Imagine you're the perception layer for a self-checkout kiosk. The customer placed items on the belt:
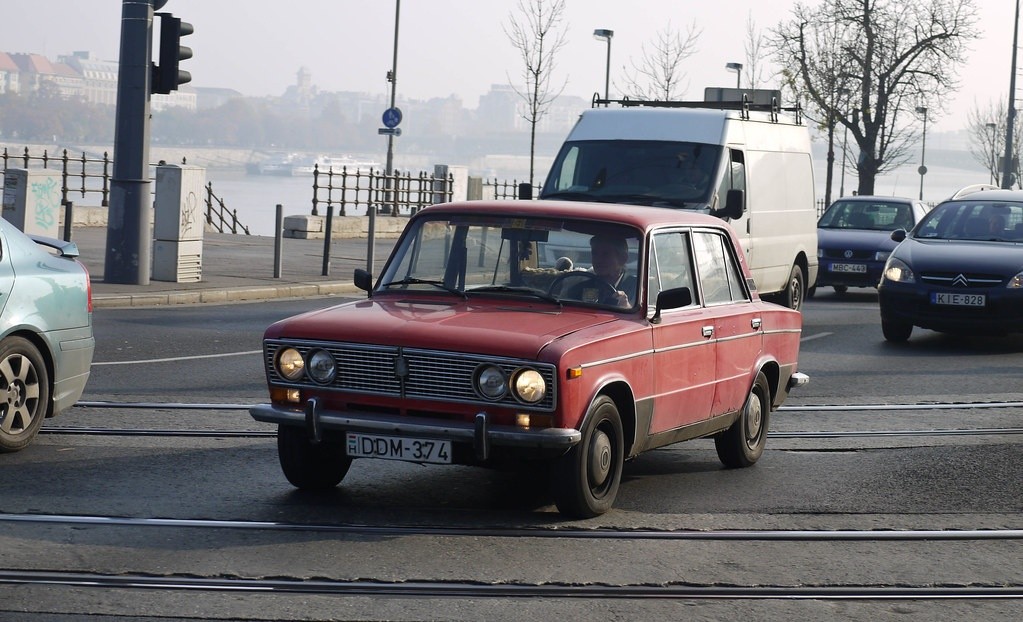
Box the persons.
[896,207,911,226]
[989,213,1005,233]
[567,235,637,308]
[685,158,711,189]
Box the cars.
[0,216,96,451]
[249,199,810,519]
[878,184,1023,342]
[817,195,931,299]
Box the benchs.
[516,266,666,308]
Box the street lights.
[984,123,997,187]
[915,105,927,198]
[594,28,614,105]
[834,87,851,195]
[724,63,744,89]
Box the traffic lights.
[154,13,193,95]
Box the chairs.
[965,218,989,238]
[846,212,869,227]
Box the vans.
[515,92,819,311]
